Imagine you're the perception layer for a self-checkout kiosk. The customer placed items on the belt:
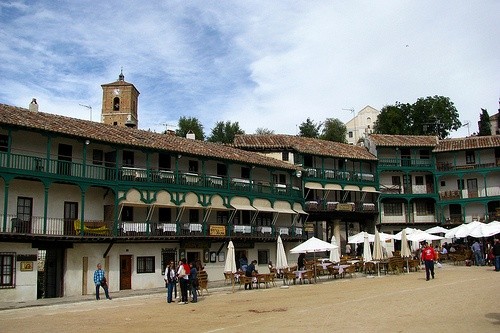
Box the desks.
[121,167,254,192]
[183,223,202,235]
[368,261,379,276]
[317,263,332,275]
[273,183,300,197]
[117,222,151,236]
[347,260,361,271]
[305,201,375,211]
[277,227,289,236]
[304,169,375,181]
[260,226,272,235]
[229,225,251,234]
[292,270,309,284]
[157,223,177,235]
[294,228,302,235]
[332,265,350,279]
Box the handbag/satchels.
[192,278,199,290]
[437,261,442,267]
[99,277,106,286]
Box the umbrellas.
[276,235,290,286]
[348,231,395,277]
[290,236,339,283]
[394,227,445,274]
[424,220,500,239]
[223,241,237,293]
[329,235,340,264]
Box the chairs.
[11,218,24,233]
[224,249,489,293]
[34,159,45,172]
[197,271,210,297]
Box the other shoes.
[190,300,197,303]
[168,300,175,303]
[426,278,429,281]
[432,275,434,278]
[178,301,188,305]
[97,297,100,300]
[107,296,111,299]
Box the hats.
[181,258,186,262]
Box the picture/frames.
[21,261,33,271]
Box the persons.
[440,234,500,272]
[421,242,438,281]
[345,243,362,256]
[296,252,308,270]
[245,260,259,290]
[93,263,111,301]
[165,257,199,305]
